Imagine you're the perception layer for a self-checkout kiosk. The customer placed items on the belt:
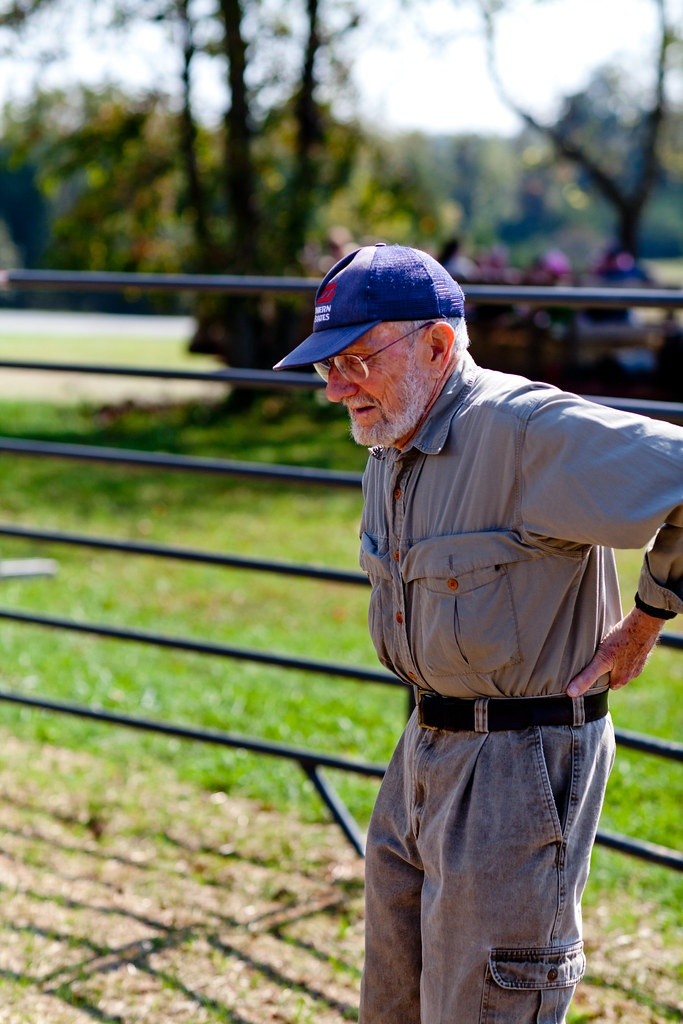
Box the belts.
[417,688,609,732]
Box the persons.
[271,242,682,1024]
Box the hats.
[272,243,464,371]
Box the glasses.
[312,321,434,384]
